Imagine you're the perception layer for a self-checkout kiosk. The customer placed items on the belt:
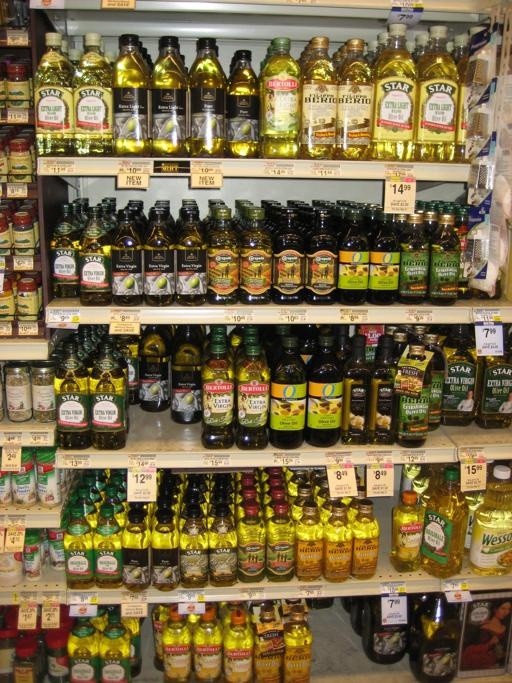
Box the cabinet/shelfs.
[1,333,66,611]
[1,8,68,339]
[36,149,512,606]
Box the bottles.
[264,488,291,522]
[422,333,444,430]
[385,324,426,364]
[33,33,74,156]
[151,36,189,158]
[389,491,424,574]
[236,502,266,583]
[88,341,126,450]
[112,34,150,157]
[272,207,305,303]
[76,468,111,528]
[408,594,461,683]
[12,638,39,683]
[0,629,18,683]
[287,470,310,506]
[234,345,270,451]
[67,617,100,683]
[223,610,254,683]
[49,204,79,299]
[323,502,352,583]
[475,324,512,429]
[254,606,284,683]
[223,601,250,628]
[121,508,151,592]
[11,451,37,507]
[278,598,308,625]
[200,332,233,362]
[263,478,287,504]
[319,491,342,523]
[348,486,366,522]
[367,335,397,444]
[352,499,379,580]
[151,468,184,528]
[68,197,468,235]
[429,214,460,305]
[19,205,40,248]
[151,604,170,662]
[176,211,206,306]
[0,277,15,322]
[449,34,468,64]
[78,206,112,308]
[162,611,192,683]
[93,504,123,589]
[0,214,12,257]
[63,507,95,590]
[48,519,67,570]
[6,63,30,109]
[336,39,375,160]
[235,334,266,364]
[206,472,235,528]
[368,211,400,305]
[31,363,56,423]
[179,504,209,589]
[107,336,130,434]
[3,362,32,422]
[206,209,239,305]
[291,484,313,522]
[179,473,210,529]
[12,211,35,256]
[412,31,429,64]
[420,467,468,578]
[208,503,237,587]
[239,207,272,304]
[54,343,89,450]
[266,503,296,583]
[469,464,512,576]
[17,278,39,322]
[416,25,460,163]
[236,474,261,503]
[0,144,8,183]
[188,37,227,158]
[200,342,235,450]
[16,132,37,177]
[192,605,223,683]
[151,508,180,592]
[99,615,131,683]
[22,622,47,676]
[340,334,371,446]
[371,25,419,162]
[112,210,143,307]
[305,334,344,447]
[22,535,42,582]
[236,489,264,521]
[73,32,113,157]
[120,615,142,678]
[30,526,47,563]
[58,39,70,60]
[361,596,406,664]
[137,325,171,413]
[0,453,13,508]
[441,323,479,428]
[455,210,472,300]
[300,35,338,161]
[315,477,328,507]
[269,336,307,451]
[170,324,202,425]
[45,630,69,683]
[295,501,323,579]
[304,210,339,305]
[144,210,175,307]
[373,30,390,62]
[259,37,300,160]
[455,25,487,163]
[399,213,429,305]
[36,449,62,507]
[224,50,259,158]
[394,345,427,448]
[263,469,286,491]
[117,334,139,404]
[73,324,129,377]
[8,138,33,183]
[284,605,312,683]
[336,214,369,305]
[104,469,127,528]
